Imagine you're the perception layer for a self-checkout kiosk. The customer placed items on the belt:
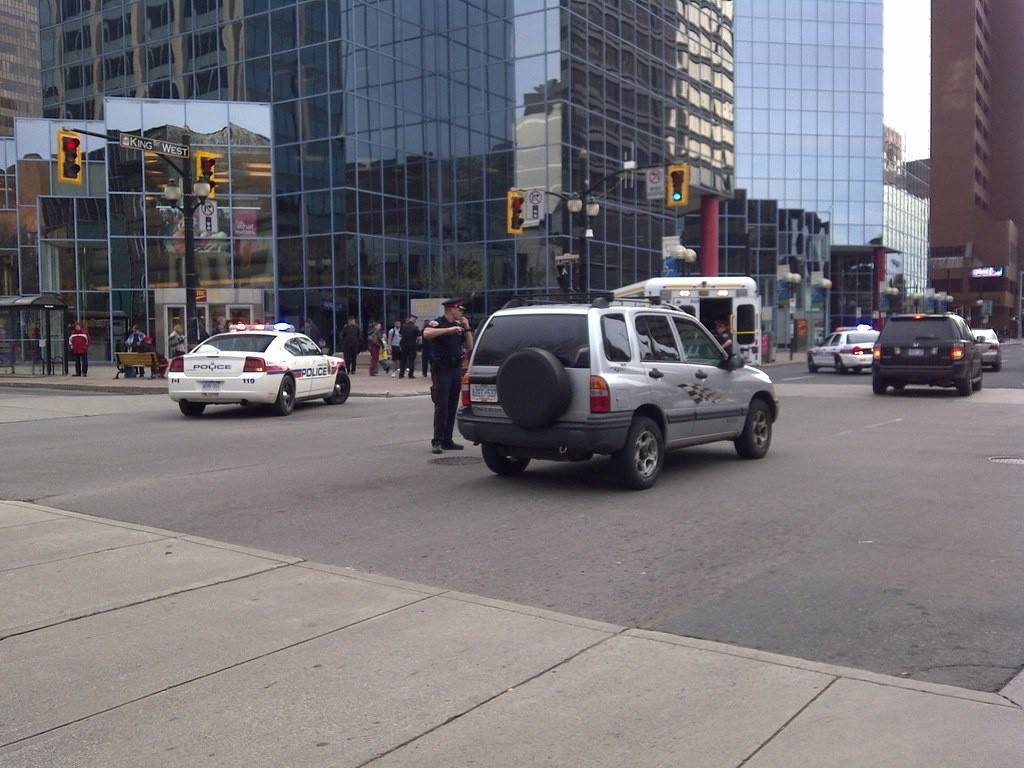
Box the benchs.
[33,356,64,376]
[113,352,164,380]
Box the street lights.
[566,192,600,304]
[784,273,801,361]
[671,245,697,276]
[913,293,922,314]
[163,175,210,353]
[933,294,942,315]
[946,295,954,311]
[819,279,832,340]
[885,287,899,315]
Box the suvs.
[872,311,986,397]
[456,292,782,490]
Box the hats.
[143,336,153,341]
[715,319,728,328]
[442,297,466,310]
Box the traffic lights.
[667,165,690,207]
[196,150,216,199]
[57,131,82,184]
[507,191,525,235]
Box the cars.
[807,327,881,376]
[168,330,351,416]
[971,329,1004,372]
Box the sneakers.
[442,441,464,450]
[432,445,442,454]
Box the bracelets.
[465,327,471,332]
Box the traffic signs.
[120,133,189,159]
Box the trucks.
[607,276,761,367]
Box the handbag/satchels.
[367,334,380,348]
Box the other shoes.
[399,376,405,379]
[409,376,415,379]
[386,367,390,373]
[347,371,350,374]
[72,374,81,376]
[351,370,355,374]
[83,374,87,377]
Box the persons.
[125,325,169,379]
[423,297,473,454]
[68,322,92,377]
[368,314,432,379]
[303,319,323,352]
[160,207,235,252]
[989,267,1001,276]
[198,316,273,345]
[340,316,363,374]
[168,324,187,358]
[716,322,733,355]
